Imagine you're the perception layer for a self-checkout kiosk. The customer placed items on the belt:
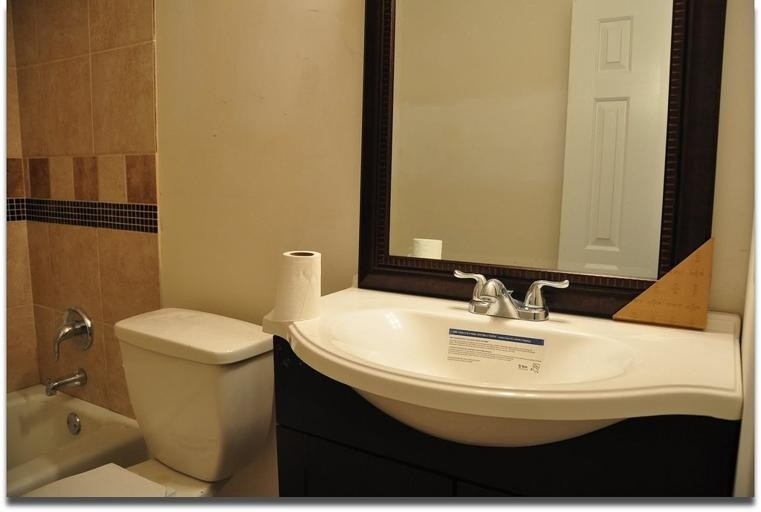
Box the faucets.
[44,368,87,397]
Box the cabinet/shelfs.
[270,346,740,499]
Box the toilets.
[22,304,276,499]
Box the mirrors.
[356,1,729,334]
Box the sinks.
[480,278,519,319]
[330,308,635,448]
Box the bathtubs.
[7,382,149,497]
